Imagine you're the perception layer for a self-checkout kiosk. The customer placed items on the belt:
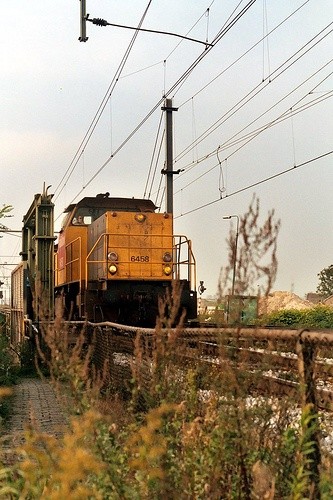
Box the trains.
[51,191,206,335]
